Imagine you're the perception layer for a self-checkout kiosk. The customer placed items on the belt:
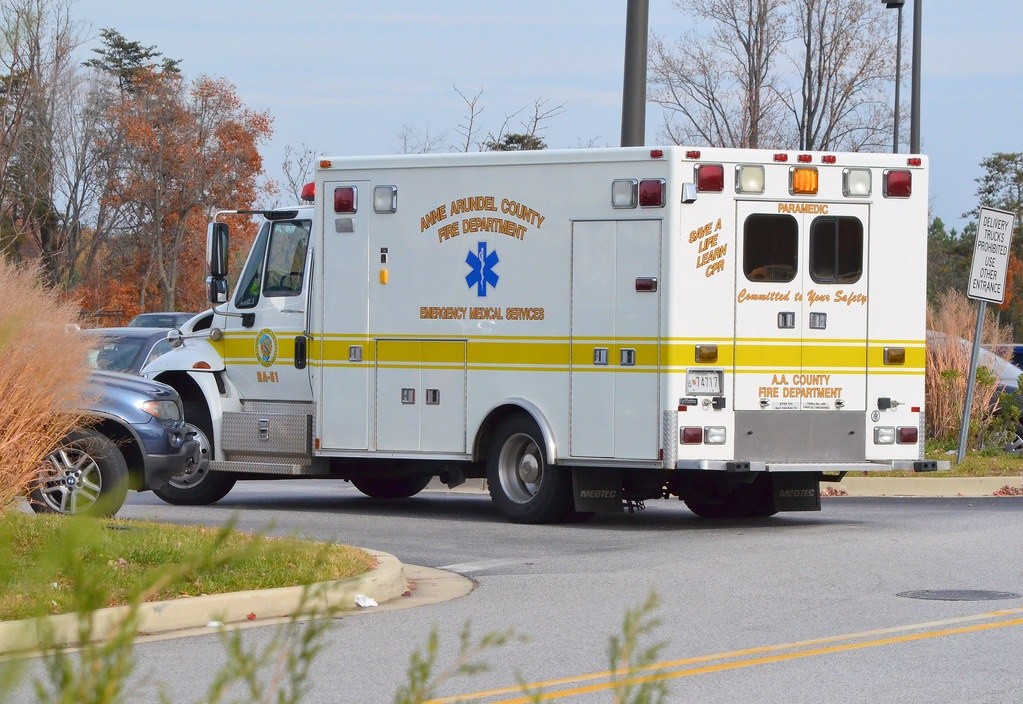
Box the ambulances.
[140,145,930,507]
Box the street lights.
[883,0,906,151]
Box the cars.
[981,344,1022,370]
[928,331,1023,455]
[132,312,200,330]
[73,326,177,377]
[26,369,190,516]
